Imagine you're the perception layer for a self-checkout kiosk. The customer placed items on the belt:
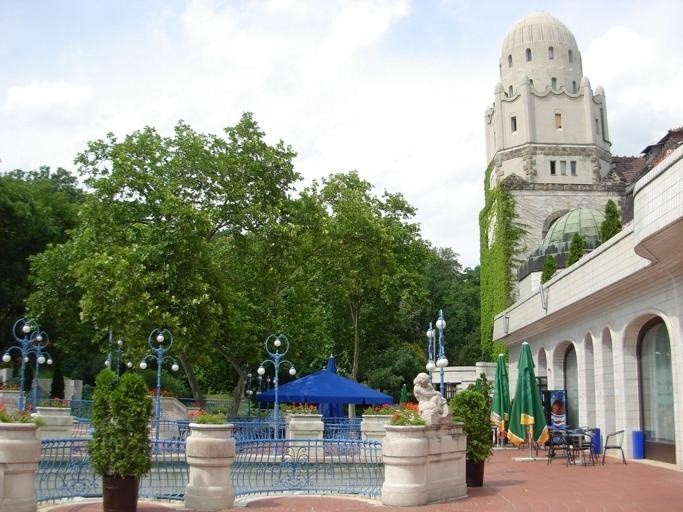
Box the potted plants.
[93,370,152,512]
[449,372,492,487]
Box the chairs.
[547,426,626,467]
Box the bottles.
[551,392,566,431]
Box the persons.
[412,372,450,423]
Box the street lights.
[138,326,180,453]
[245,368,253,417]
[434,307,449,397]
[424,319,438,383]
[256,331,296,455]
[23,329,54,408]
[0,317,46,413]
[101,338,134,376]
[265,373,271,414]
[255,375,263,415]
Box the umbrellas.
[397,382,408,404]
[253,367,394,415]
[313,351,347,437]
[475,377,481,392]
[506,340,551,462]
[490,351,518,451]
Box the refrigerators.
[540,389,567,445]
